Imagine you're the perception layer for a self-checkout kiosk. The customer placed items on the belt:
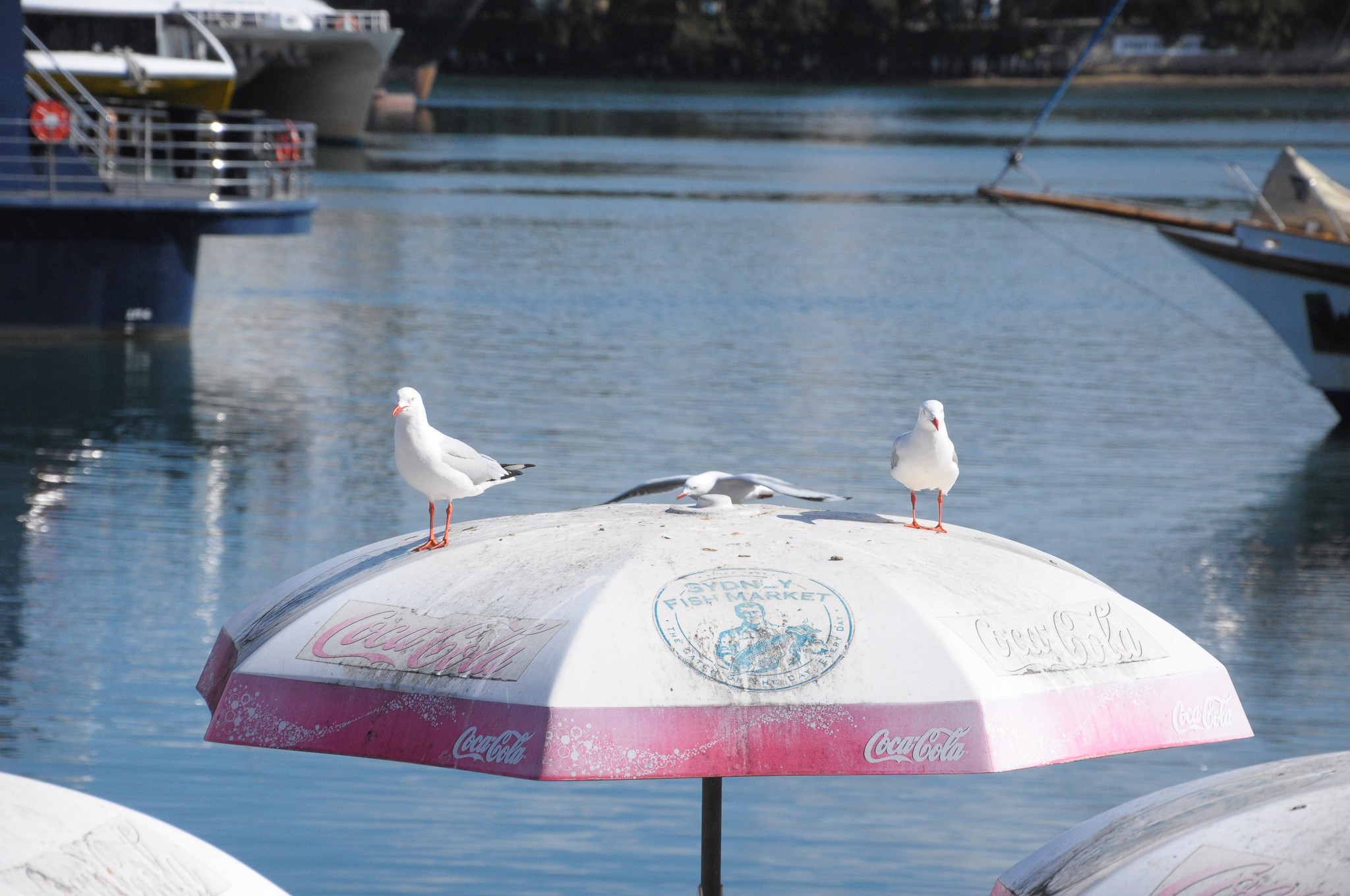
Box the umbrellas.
[197,497,1254,896]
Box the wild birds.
[597,471,852,503]
[392,387,536,551]
[890,401,960,532]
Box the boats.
[20,0,406,151]
[979,0,1350,446]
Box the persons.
[267,109,300,200]
